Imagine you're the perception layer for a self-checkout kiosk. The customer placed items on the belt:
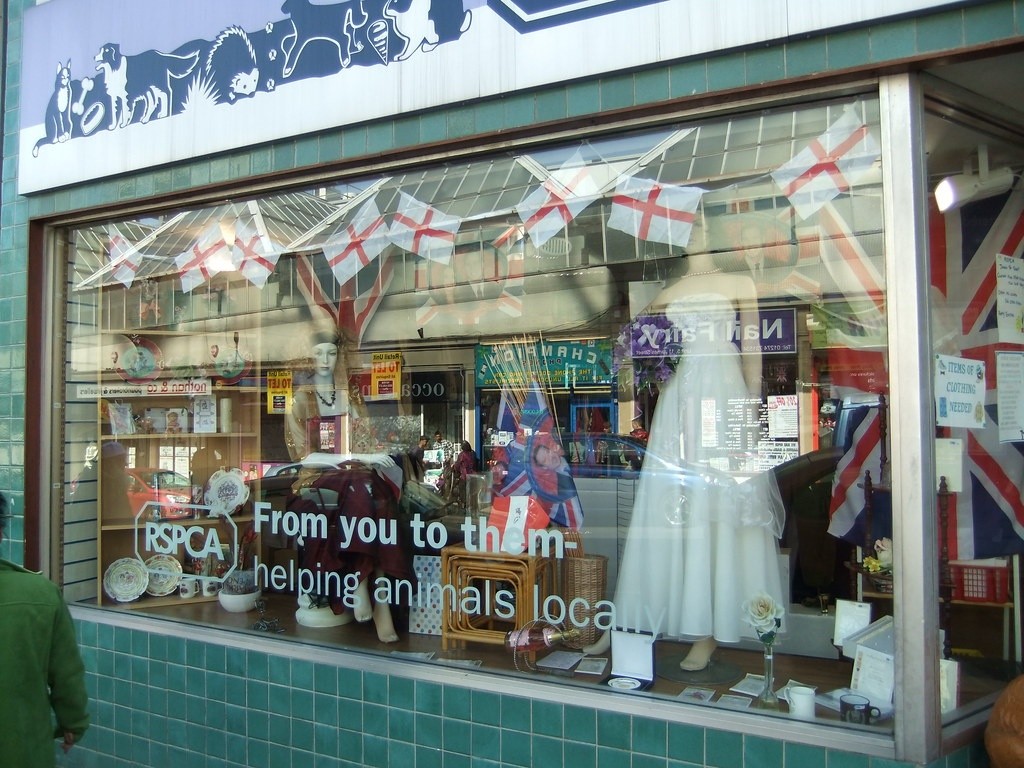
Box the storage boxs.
[948,561,1010,603]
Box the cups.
[840,694,881,726]
[203,577,223,597]
[179,577,199,599]
[784,686,816,718]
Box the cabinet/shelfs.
[95,238,260,608]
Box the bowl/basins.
[218,588,262,613]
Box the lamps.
[933,143,1015,214]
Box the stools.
[441,541,558,665]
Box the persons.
[581,200,789,670]
[437,248,503,312]
[269,318,402,645]
[432,431,454,468]
[534,443,578,498]
[572,416,649,472]
[491,461,508,485]
[409,436,428,465]
[0,494,89,768]
[452,440,479,506]
[720,223,786,282]
[166,412,181,435]
[71,442,134,539]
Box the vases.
[759,648,778,703]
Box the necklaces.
[316,377,336,406]
[681,267,722,277]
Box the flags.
[174,223,235,294]
[501,382,585,530]
[814,191,1023,562]
[411,224,524,324]
[106,223,142,289]
[606,174,702,248]
[231,220,284,289]
[515,151,603,248]
[385,194,462,266]
[322,199,392,286]
[488,395,518,505]
[769,110,881,221]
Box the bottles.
[504,627,582,654]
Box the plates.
[144,553,183,596]
[203,468,250,517]
[103,557,148,602]
[112,336,165,387]
[204,341,253,384]
[608,677,640,691]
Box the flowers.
[740,595,784,643]
[613,313,683,386]
